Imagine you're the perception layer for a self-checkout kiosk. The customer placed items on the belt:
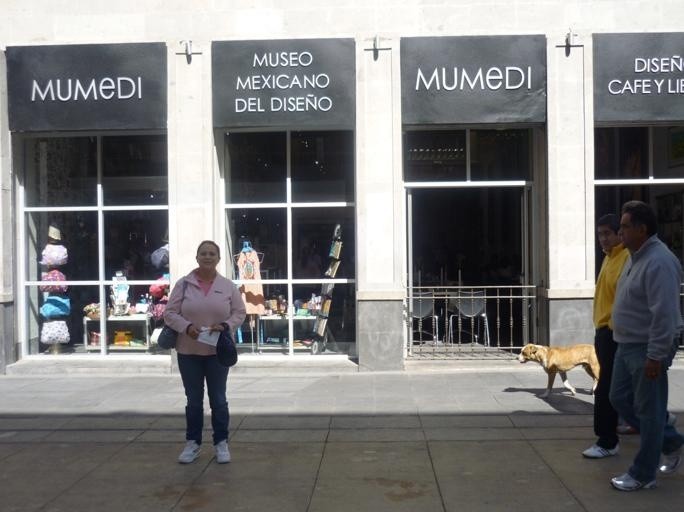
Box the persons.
[581,215,678,458]
[608,199,683,492]
[163,240,247,465]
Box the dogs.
[516,342,602,401]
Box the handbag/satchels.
[151,244,169,344]
[217,324,237,366]
[39,245,70,343]
[159,325,178,349]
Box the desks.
[256,312,318,354]
[82,312,154,354]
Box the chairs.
[412,292,439,347]
[448,290,490,347]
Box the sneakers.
[611,473,655,492]
[660,447,681,474]
[582,445,618,458]
[667,414,677,428]
[214,439,230,463]
[179,440,202,463]
